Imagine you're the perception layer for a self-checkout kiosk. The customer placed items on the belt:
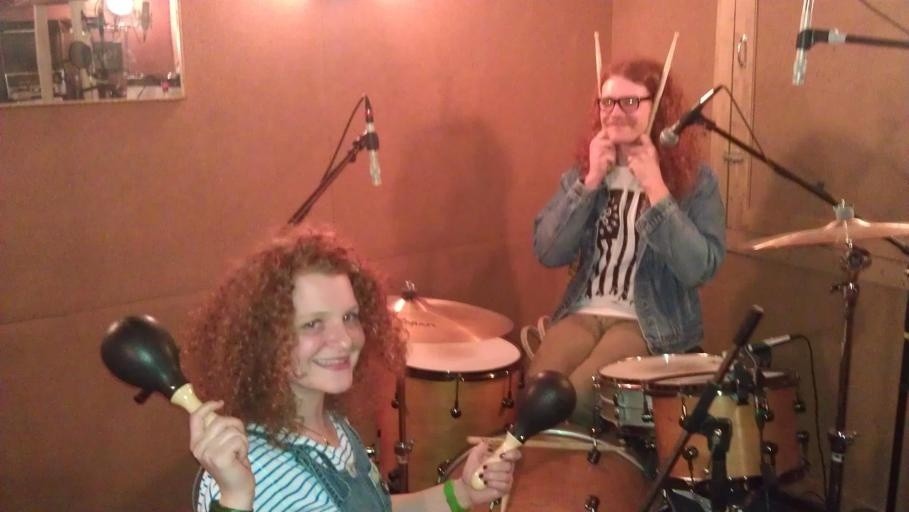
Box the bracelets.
[442,479,467,512]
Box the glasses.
[595,94,652,112]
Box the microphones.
[791,0,815,87]
[364,94,383,187]
[747,334,803,352]
[659,83,724,149]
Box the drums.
[651,371,802,486]
[438,425,674,512]
[379,338,522,495]
[593,353,734,448]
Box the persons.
[514,53,730,439]
[180,227,522,512]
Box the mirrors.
[1,0,184,108]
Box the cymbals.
[384,296,513,342]
[745,216,903,250]
